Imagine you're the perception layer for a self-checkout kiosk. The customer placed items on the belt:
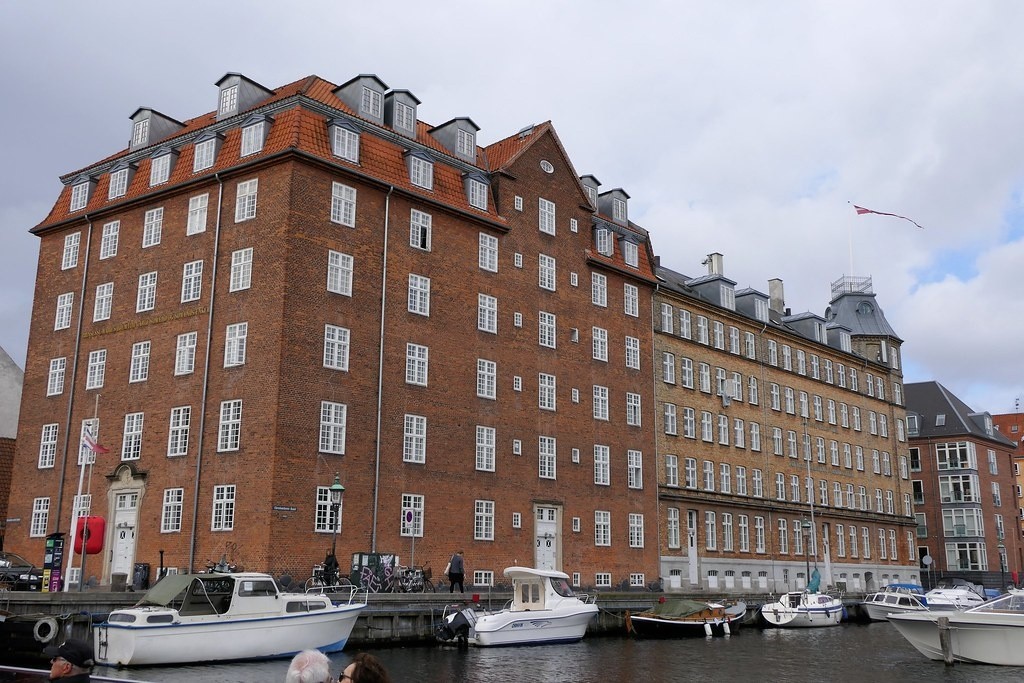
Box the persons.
[43,638,94,683]
[336,653,392,683]
[448,550,466,593]
[320,548,341,593]
[286,648,334,683]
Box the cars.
[0,551,64,592]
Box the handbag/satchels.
[443,555,454,576]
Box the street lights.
[801,515,812,587]
[327,471,346,555]
[996,538,1007,594]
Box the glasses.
[338,672,354,682]
[49,655,65,662]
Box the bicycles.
[408,561,436,593]
[193,559,236,592]
[304,564,353,593]
[392,564,425,593]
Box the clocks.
[859,302,872,315]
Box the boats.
[90,573,368,664]
[0,608,117,658]
[925,577,985,611]
[885,588,1024,666]
[436,566,599,646]
[626,600,747,635]
[762,588,849,626]
[858,584,930,620]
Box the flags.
[82,427,110,454]
[853,204,925,228]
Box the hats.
[42,638,96,669]
